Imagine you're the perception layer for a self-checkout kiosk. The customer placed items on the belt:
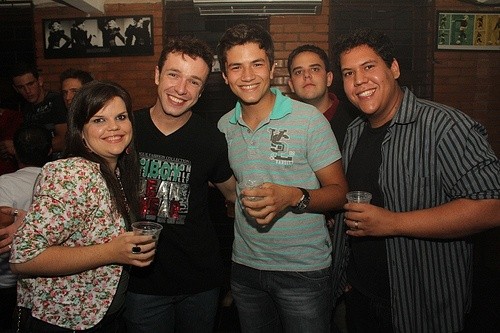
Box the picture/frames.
[437,12,500,51]
[42,14,154,59]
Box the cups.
[131,221,163,261]
[238,178,264,219]
[346,191,372,230]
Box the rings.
[133,243,141,254]
[355,220,359,229]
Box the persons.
[46,14,154,51]
[212,22,351,331]
[0,118,53,328]
[9,63,67,170]
[117,36,243,332]
[8,81,154,332]
[328,28,499,332]
[287,45,361,164]
[55,68,96,156]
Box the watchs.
[291,185,312,213]
